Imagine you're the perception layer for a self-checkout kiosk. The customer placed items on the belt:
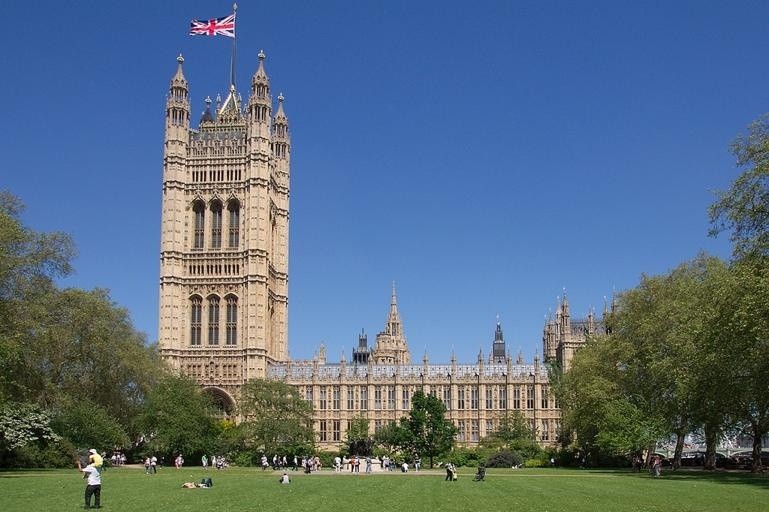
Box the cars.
[651,451,769,466]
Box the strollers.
[472,463,485,481]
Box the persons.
[77,454,102,510]
[652,455,661,477]
[149,455,158,474]
[202,454,229,470]
[452,467,457,481]
[142,455,150,474]
[278,473,292,485]
[175,453,185,472]
[85,449,103,474]
[180,477,212,489]
[110,452,128,465]
[445,458,452,481]
[261,452,423,475]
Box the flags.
[188,15,234,38]
